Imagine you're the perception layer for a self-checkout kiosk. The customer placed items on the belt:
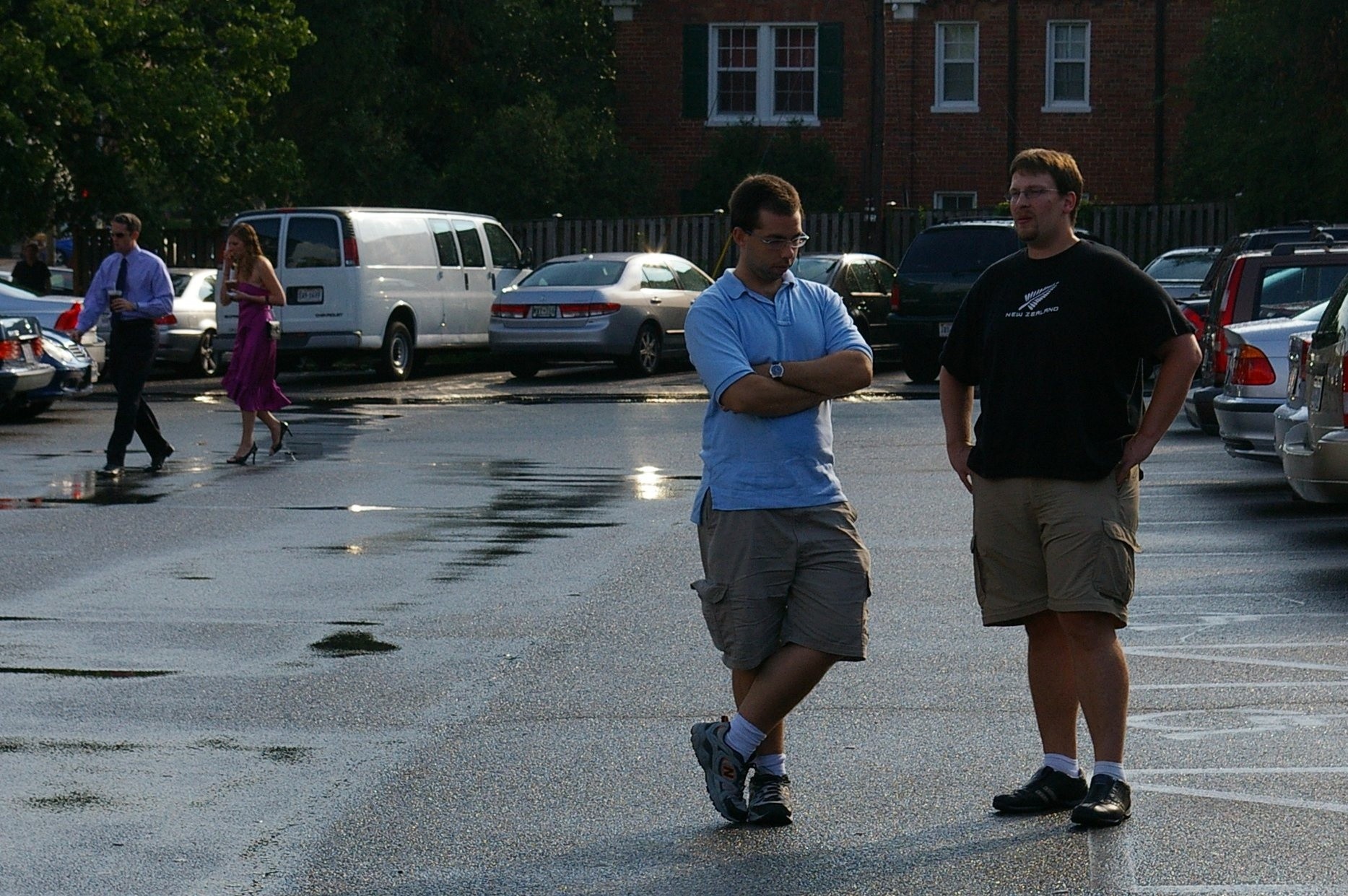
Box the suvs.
[1140,220,1348,505]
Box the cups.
[225,280,236,292]
[107,290,122,312]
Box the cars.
[789,252,898,366]
[488,252,715,378]
[0,265,112,422]
[153,268,219,377]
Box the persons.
[220,223,293,464]
[70,214,175,477]
[684,174,874,824]
[11,233,51,294]
[939,149,1203,824]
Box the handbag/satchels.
[266,296,281,340]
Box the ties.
[111,258,127,331]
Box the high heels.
[268,421,293,456]
[226,441,257,465]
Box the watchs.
[769,361,783,381]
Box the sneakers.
[745,774,794,826]
[1070,774,1133,827]
[690,715,755,822]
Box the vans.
[212,207,534,381]
[886,219,1106,383]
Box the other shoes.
[95,463,125,478]
[146,444,173,473]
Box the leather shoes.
[992,764,1088,817]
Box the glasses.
[740,230,810,252]
[110,230,131,239]
[1003,184,1062,199]
